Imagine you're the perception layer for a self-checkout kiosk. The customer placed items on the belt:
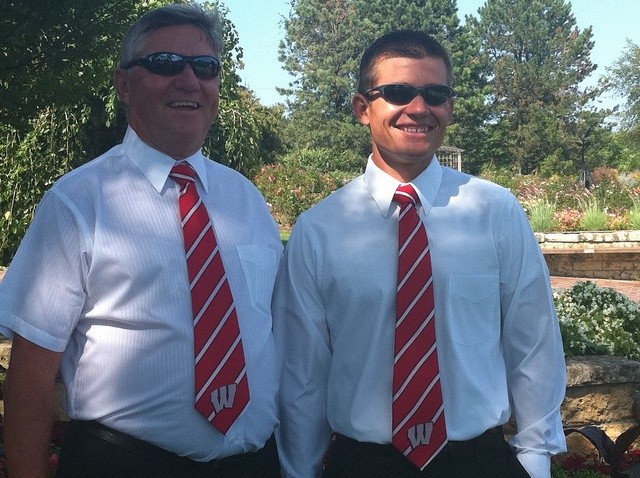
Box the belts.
[336,427,504,460]
[83,420,269,478]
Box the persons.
[1,5,285,476]
[269,28,569,478]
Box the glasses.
[120,51,222,79]
[363,82,456,106]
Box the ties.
[169,164,250,435]
[392,184,448,470]
